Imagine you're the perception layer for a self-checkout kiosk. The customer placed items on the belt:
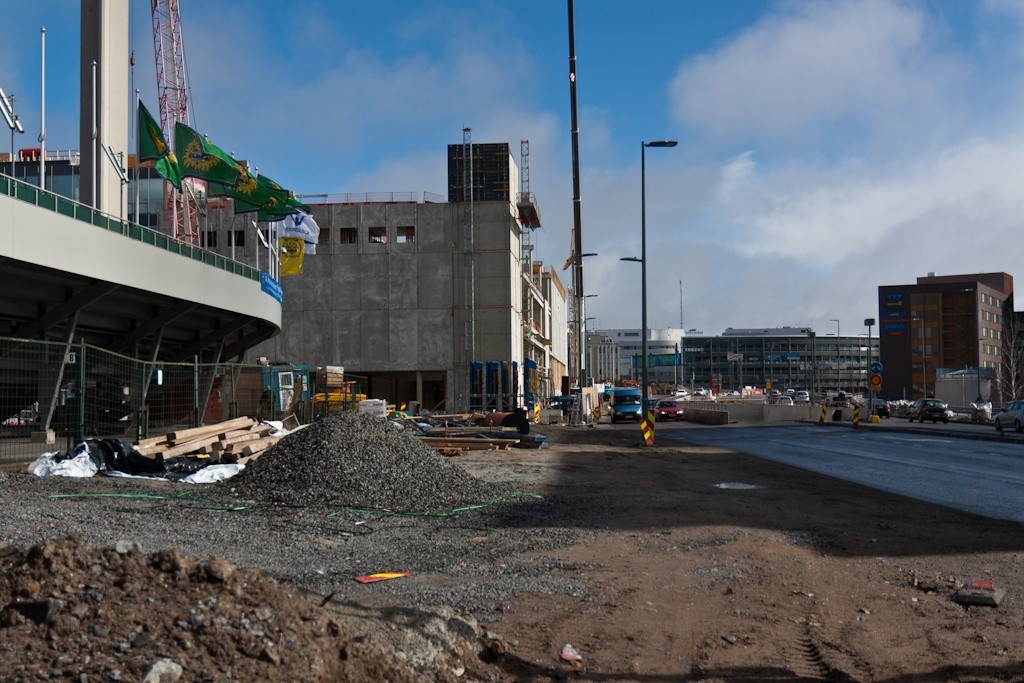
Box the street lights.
[829,319,841,397]
[705,338,713,374]
[620,137,680,439]
[960,287,981,400]
[808,332,816,407]
[863,318,876,415]
[913,316,927,399]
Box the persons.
[974,392,986,404]
[928,391,935,398]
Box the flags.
[138,98,181,190]
[236,165,320,277]
[175,120,241,187]
[207,139,277,208]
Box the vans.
[607,385,643,424]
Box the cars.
[671,389,677,396]
[906,398,949,424]
[866,399,891,419]
[992,400,1024,434]
[675,388,687,397]
[768,388,855,411]
[695,387,707,397]
[653,399,686,422]
